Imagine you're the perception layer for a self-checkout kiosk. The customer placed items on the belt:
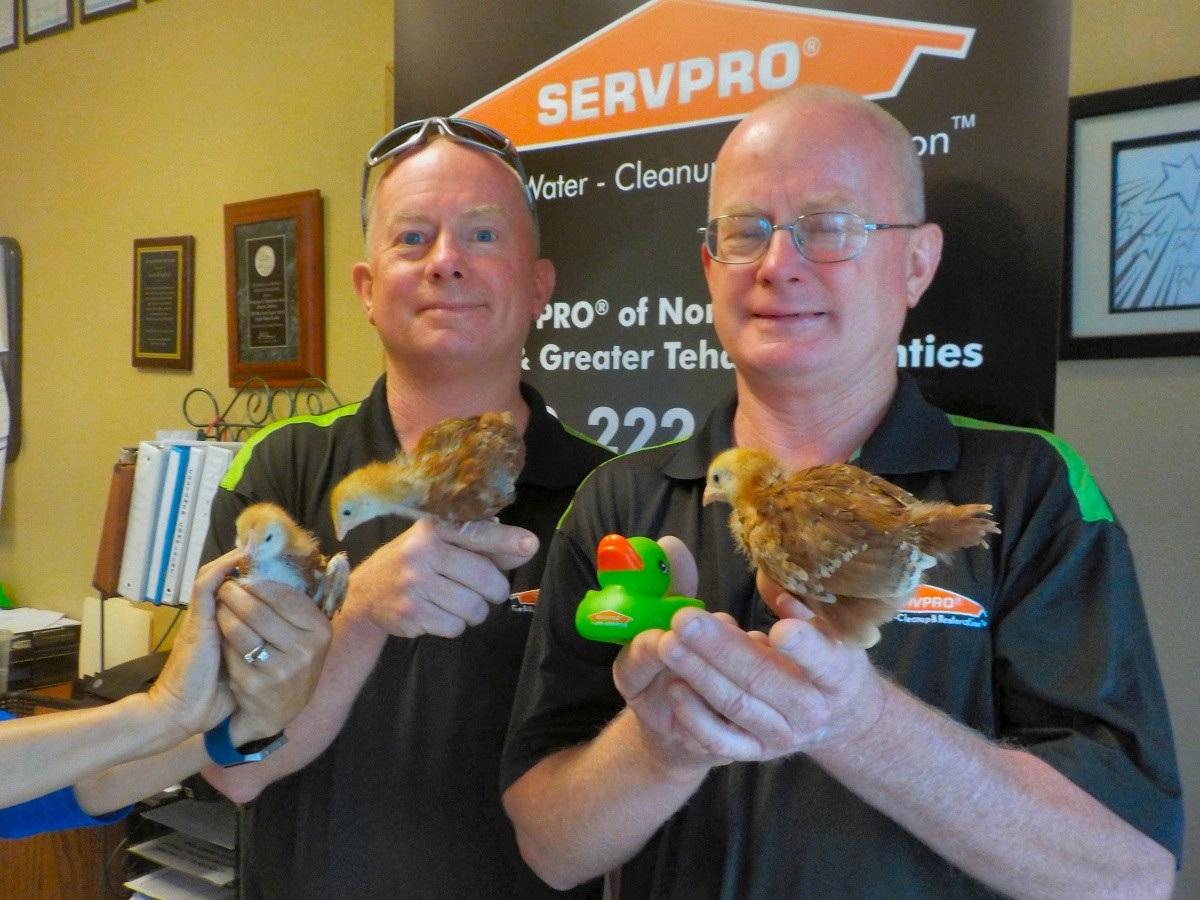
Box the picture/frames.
[1058,75,1200,359]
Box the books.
[93,435,249,607]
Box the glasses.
[359,115,539,243]
[695,211,918,264]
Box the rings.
[243,641,269,664]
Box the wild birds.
[234,501,350,618]
[701,448,1001,605]
[332,410,524,542]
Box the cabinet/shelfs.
[122,798,258,900]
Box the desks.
[0,682,132,900]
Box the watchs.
[204,714,290,768]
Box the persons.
[501,85,1184,900]
[0,548,333,838]
[197,116,623,900]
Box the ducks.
[575,535,701,649]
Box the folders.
[178,442,247,607]
[115,441,171,605]
